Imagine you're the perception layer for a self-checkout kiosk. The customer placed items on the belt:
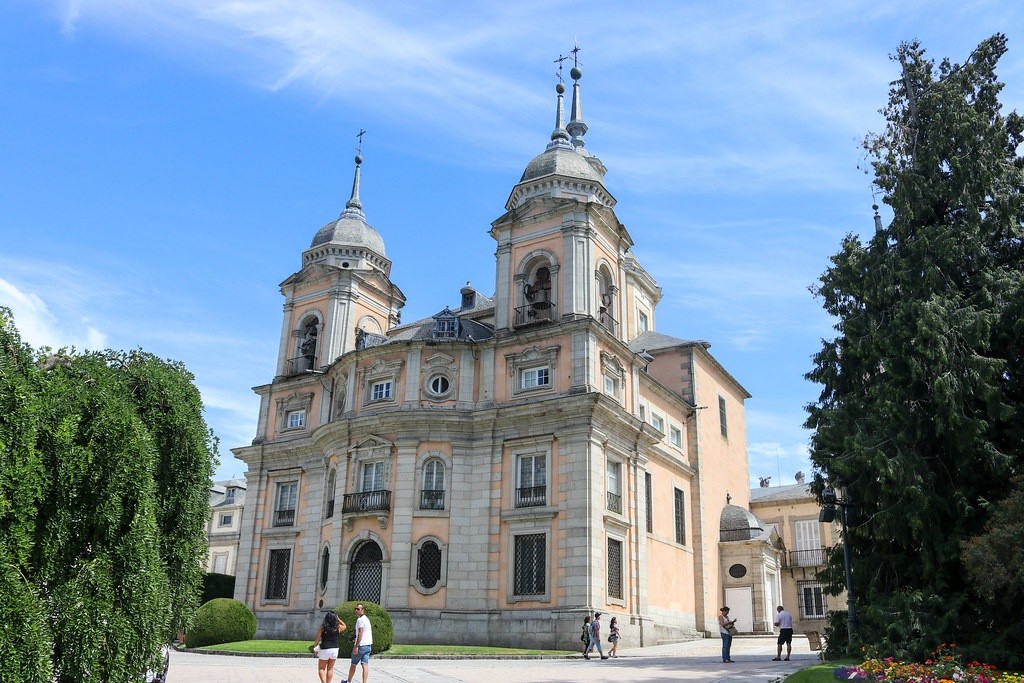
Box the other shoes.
[724,659,735,663]
[784,658,789,661]
[772,657,781,661]
[608,652,611,657]
[584,654,590,660]
[601,656,609,659]
[613,655,618,658]
[341,680,347,683]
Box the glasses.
[355,608,360,610]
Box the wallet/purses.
[313,644,321,657]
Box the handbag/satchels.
[581,634,585,641]
[608,636,612,642]
[727,626,738,636]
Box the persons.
[582,616,591,655]
[607,617,621,658]
[772,606,793,661]
[314,611,346,683]
[341,603,372,683]
[719,607,737,662]
[584,612,609,660]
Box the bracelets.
[355,645,360,647]
[338,620,339,621]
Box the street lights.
[818,485,855,649]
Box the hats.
[595,612,601,617]
